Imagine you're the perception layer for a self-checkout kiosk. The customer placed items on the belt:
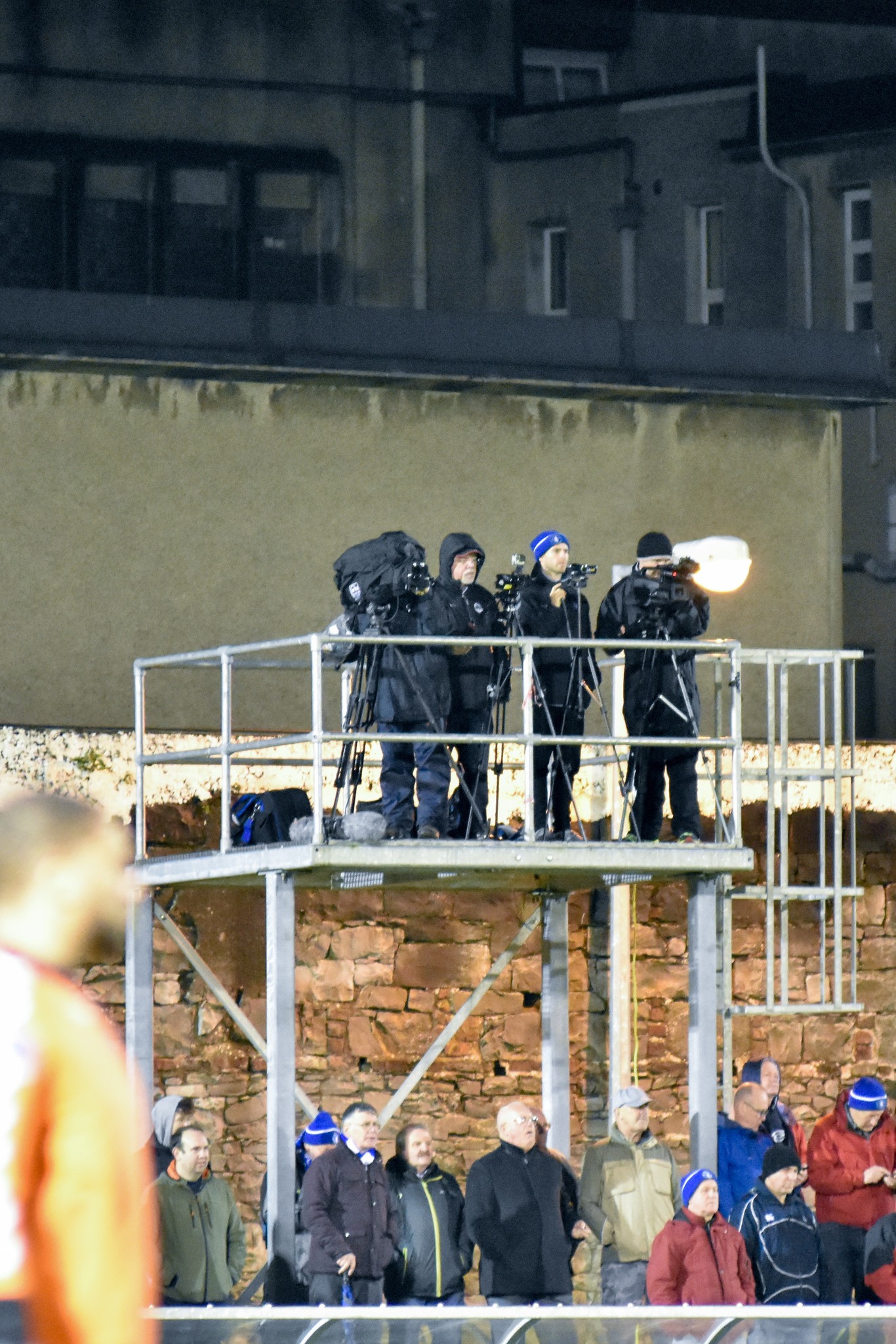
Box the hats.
[636,532,672,561]
[530,530,570,563]
[762,1143,802,1178]
[303,1110,341,1146]
[681,1170,718,1208]
[847,1077,888,1111]
[456,548,483,557]
[613,1087,653,1112]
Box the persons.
[513,531,602,842]
[367,531,509,839]
[594,531,714,843]
[145,1056,896,1344]
[0,796,162,1344]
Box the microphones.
[288,809,388,844]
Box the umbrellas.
[342,1268,356,1343]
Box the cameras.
[569,563,597,575]
[496,554,533,590]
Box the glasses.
[501,1115,539,1129]
[744,1101,769,1118]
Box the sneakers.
[622,832,659,843]
[676,832,701,843]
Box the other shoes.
[418,825,439,839]
[384,825,406,839]
[535,828,554,840]
[548,830,584,841]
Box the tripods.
[326,574,731,845]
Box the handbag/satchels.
[230,787,312,847]
